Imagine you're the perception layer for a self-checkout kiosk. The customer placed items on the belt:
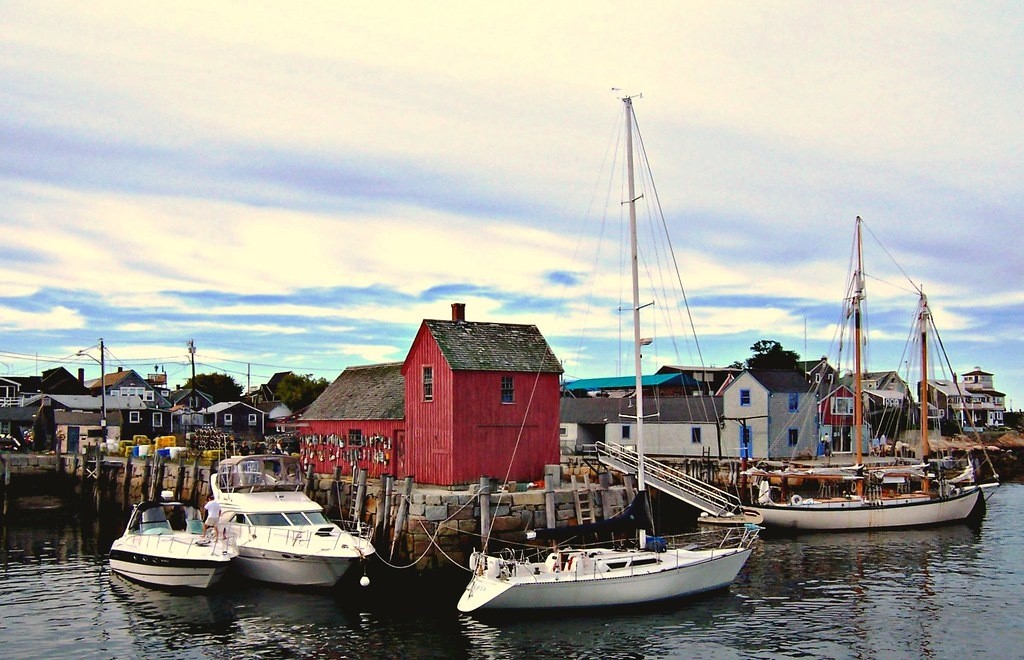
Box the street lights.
[76,353,107,459]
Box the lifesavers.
[851,482,857,493]
[792,494,803,507]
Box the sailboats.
[738,216,1008,534]
[453,88,760,613]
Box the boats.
[108,490,237,590]
[211,454,376,588]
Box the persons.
[872,434,903,456]
[199,495,221,543]
[227,431,300,479]
[822,432,833,457]
